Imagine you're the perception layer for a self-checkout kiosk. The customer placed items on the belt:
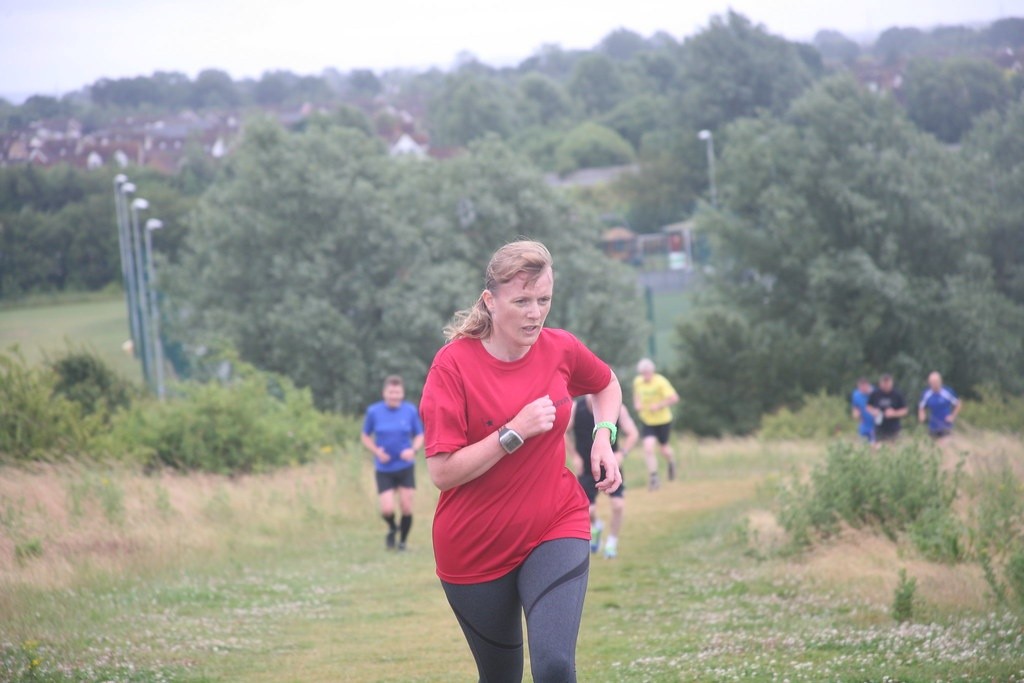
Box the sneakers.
[605,534,618,558]
[650,472,659,490]
[399,542,406,549]
[387,526,398,545]
[669,461,676,480]
[590,520,602,553]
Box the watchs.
[499,425,524,455]
[590,423,618,445]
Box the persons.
[360,375,424,550]
[564,388,638,556]
[632,358,677,488]
[417,240,625,683]
[845,370,959,440]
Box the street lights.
[115,171,133,306]
[696,128,721,214]
[118,180,141,368]
[129,195,156,400]
[143,217,166,408]
